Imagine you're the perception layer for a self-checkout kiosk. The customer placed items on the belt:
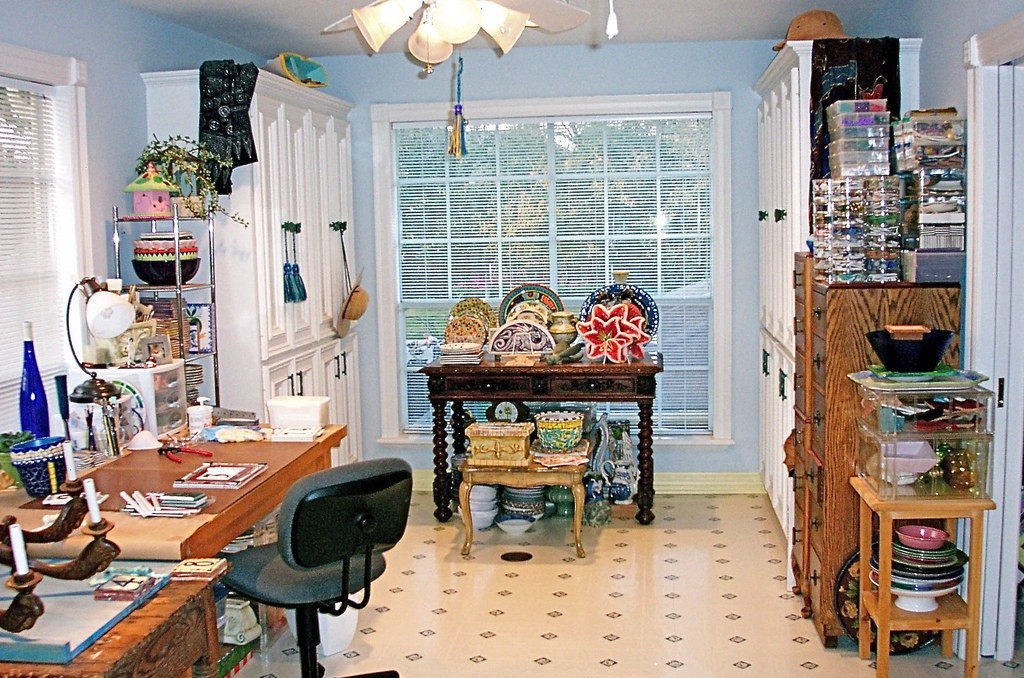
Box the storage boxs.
[828,111,893,130]
[827,99,887,118]
[904,139,965,170]
[830,124,890,141]
[829,151,889,167]
[829,162,890,176]
[893,144,907,173]
[891,121,904,145]
[903,118,965,141]
[829,136,888,155]
[465,423,535,460]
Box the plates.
[826,364,987,654]
[437,282,658,366]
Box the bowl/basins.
[131,232,200,286]
[8,435,69,498]
[458,412,585,538]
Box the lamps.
[65,275,134,400]
[0,440,121,632]
[353,1,539,75]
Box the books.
[121,491,207,518]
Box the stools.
[457,456,588,559]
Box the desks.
[0,422,351,678]
[417,351,658,522]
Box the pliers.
[157,446,213,462]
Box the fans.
[321,1,591,33]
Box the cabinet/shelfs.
[141,68,361,466]
[760,43,993,678]
[112,201,219,409]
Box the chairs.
[222,458,411,677]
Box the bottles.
[18,322,50,437]
